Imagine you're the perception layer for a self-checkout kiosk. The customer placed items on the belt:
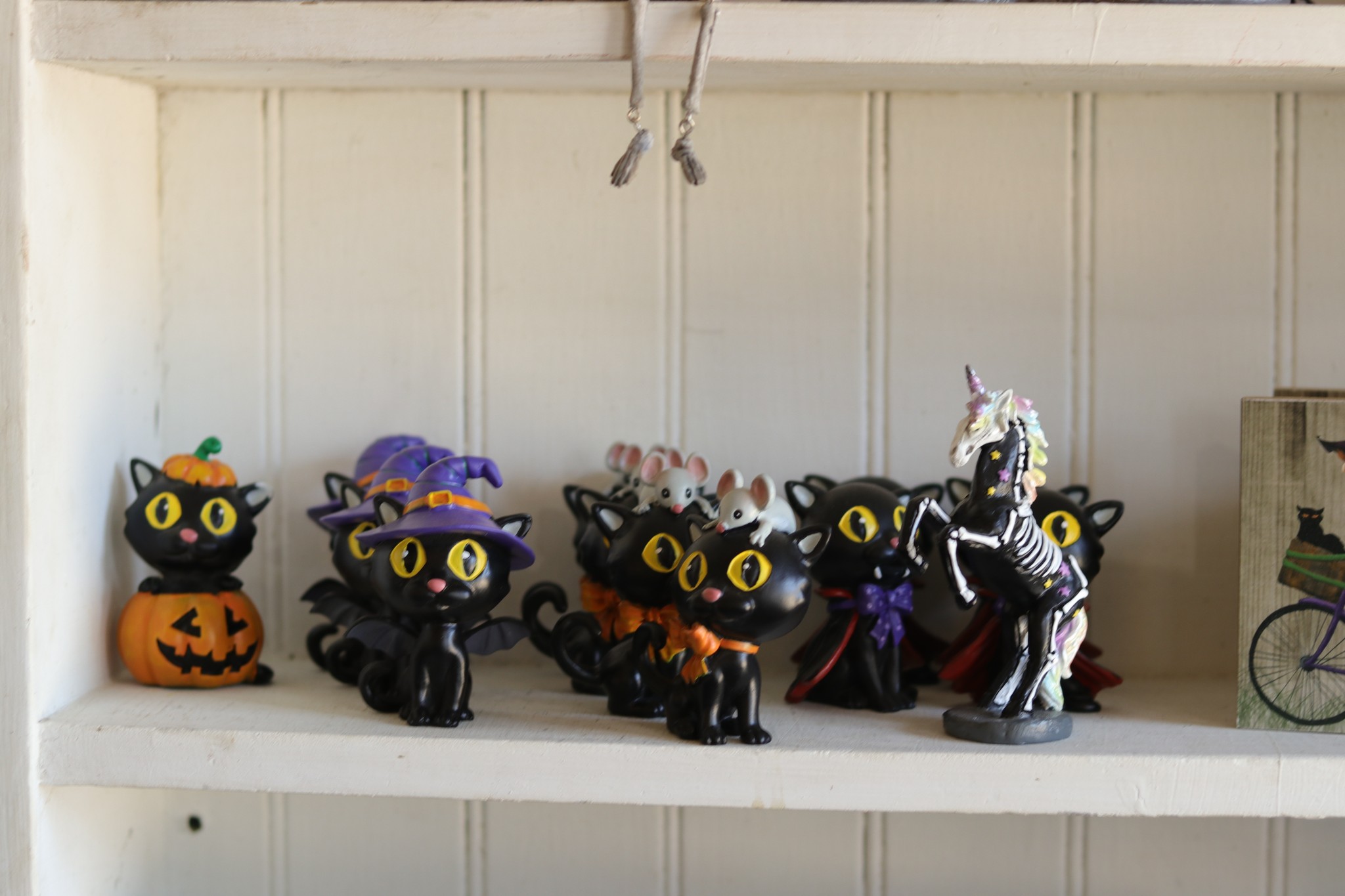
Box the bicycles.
[1247,537,1345,726]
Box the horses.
[899,362,1092,722]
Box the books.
[1233,387,1345,734]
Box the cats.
[103,436,1130,747]
